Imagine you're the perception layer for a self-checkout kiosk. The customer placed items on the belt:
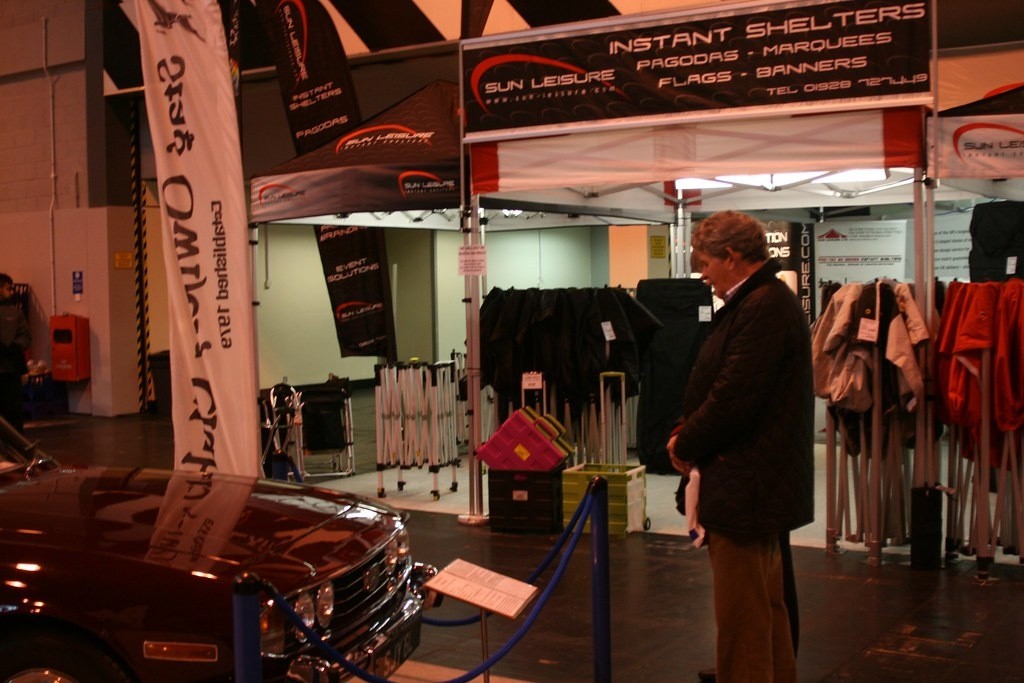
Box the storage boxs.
[471,405,651,539]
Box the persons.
[0,272,31,426]
[667,210,814,682]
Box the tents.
[471,104,931,519]
[810,276,931,566]
[248,159,474,516]
[927,82,1024,563]
[458,286,664,465]
[938,277,1024,581]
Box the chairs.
[261,373,357,480]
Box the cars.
[0,418,444,683]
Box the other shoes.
[698,669,716,683]
